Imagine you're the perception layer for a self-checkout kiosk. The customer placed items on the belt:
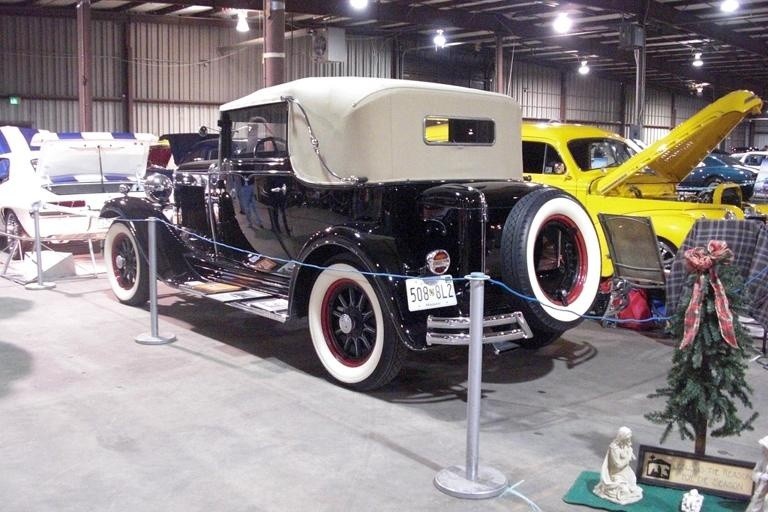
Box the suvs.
[99,76,604,392]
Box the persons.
[593,425,643,506]
[230,171,291,237]
[747,435,768,512]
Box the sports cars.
[0,124,160,260]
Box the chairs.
[596,212,671,328]
